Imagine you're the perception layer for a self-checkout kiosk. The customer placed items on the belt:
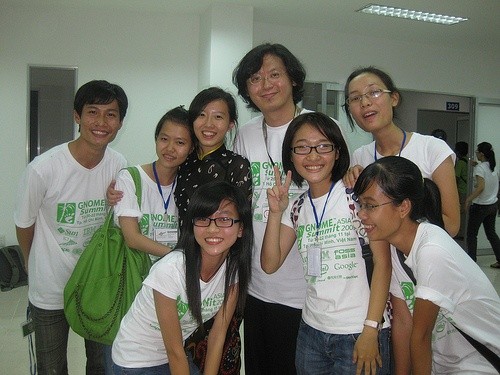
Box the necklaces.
[199,270,219,283]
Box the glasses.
[475,150,479,153]
[245,71,287,85]
[290,144,337,155]
[192,216,240,228]
[352,195,415,213]
[345,88,392,105]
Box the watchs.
[364,319,382,333]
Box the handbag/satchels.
[64,167,152,346]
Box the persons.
[109,180,254,375]
[105,87,251,375]
[340,66,460,239]
[228,43,344,375]
[103,105,195,375]
[14,80,128,375]
[260,112,391,375]
[431,130,500,268]
[352,156,500,375]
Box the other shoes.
[491,262,500,268]
